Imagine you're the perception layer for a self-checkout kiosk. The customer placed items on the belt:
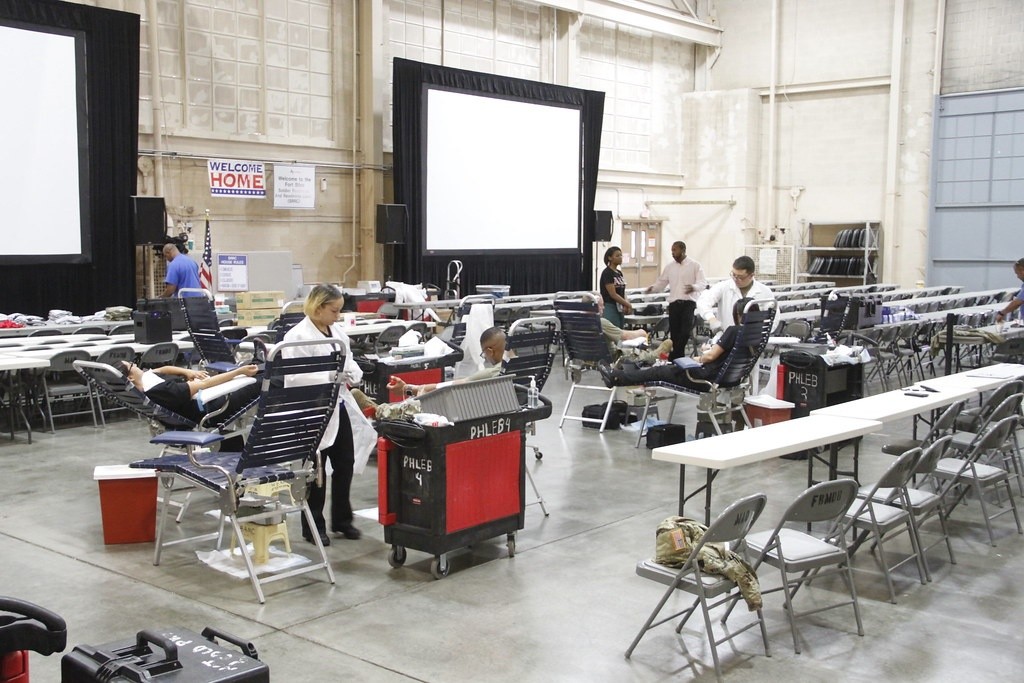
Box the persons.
[582,291,673,367]
[598,297,763,392]
[106,360,263,429]
[997,257,1024,323]
[600,247,632,329]
[697,256,780,333]
[156,244,204,297]
[281,285,378,546]
[350,326,517,419]
[645,241,707,365]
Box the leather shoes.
[332,524,360,539]
[305,532,330,546]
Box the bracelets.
[998,311,1003,316]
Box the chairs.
[0,280,1024,683]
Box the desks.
[0,357,51,445]
[914,363,1024,440]
[651,413,882,541]
[808,385,978,488]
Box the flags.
[198,217,213,292]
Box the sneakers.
[351,389,378,421]
[655,339,673,357]
[252,337,267,363]
[598,358,617,389]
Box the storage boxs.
[740,395,795,428]
[93,465,158,545]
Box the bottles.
[880,306,915,323]
[528,380,539,409]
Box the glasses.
[480,346,492,358]
[730,270,752,282]
[128,361,134,374]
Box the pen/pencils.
[904,381,940,398]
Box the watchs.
[407,386,413,395]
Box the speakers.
[377,204,405,244]
[131,196,165,245]
[592,210,612,242]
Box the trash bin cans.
[93,461,159,545]
[745,394,796,430]
